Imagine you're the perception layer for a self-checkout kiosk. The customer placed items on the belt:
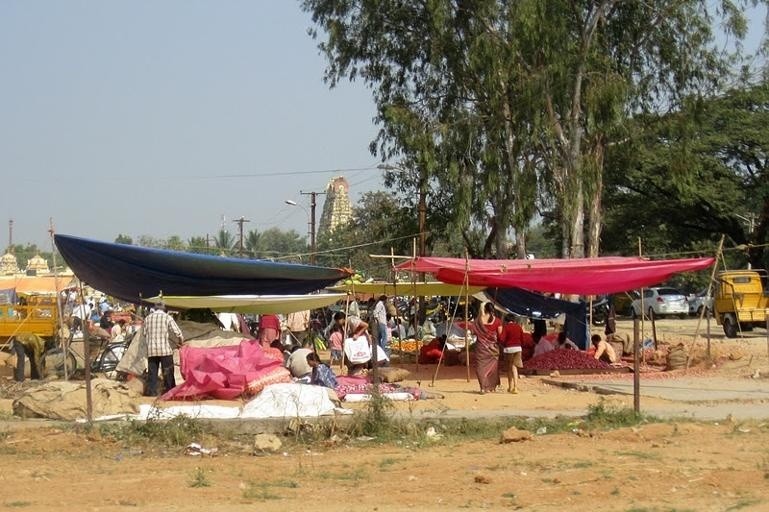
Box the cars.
[630,288,715,320]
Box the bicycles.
[37,318,131,380]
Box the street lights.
[220,213,229,260]
[377,162,447,326]
[284,199,310,265]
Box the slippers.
[479,387,520,395]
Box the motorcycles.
[578,296,611,329]
[390,296,562,322]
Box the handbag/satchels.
[331,384,345,400]
[381,327,391,343]
[343,335,371,364]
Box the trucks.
[0,296,60,358]
[713,269,768,337]
